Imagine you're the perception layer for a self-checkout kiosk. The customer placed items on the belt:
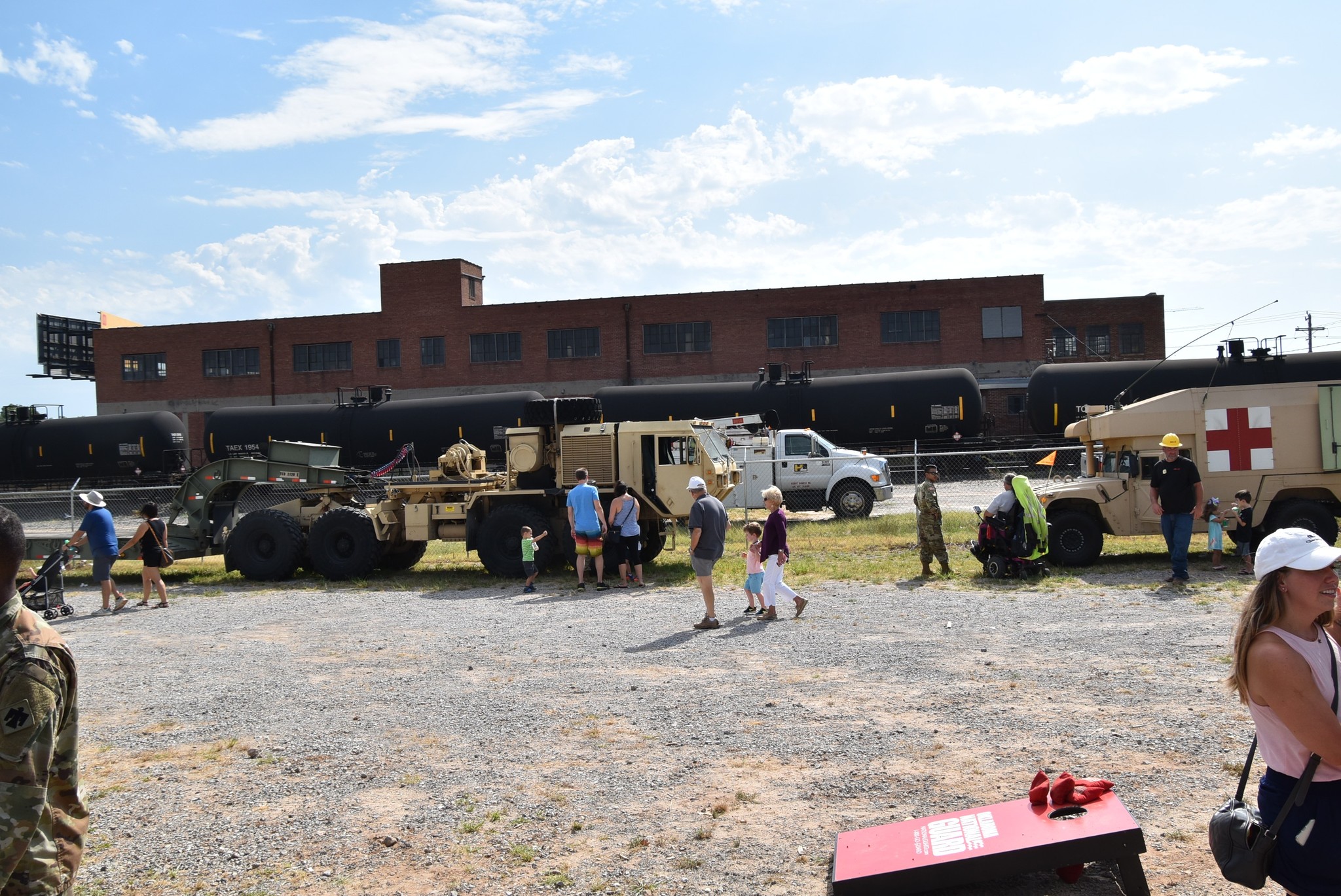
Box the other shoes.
[922,569,935,575]
[1164,574,1189,582]
[971,539,989,562]
[524,586,537,593]
[942,568,954,575]
[1212,565,1227,569]
[577,583,586,592]
[597,582,607,590]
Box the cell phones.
[776,552,789,567]
[974,506,982,513]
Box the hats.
[1254,527,1341,581]
[79,491,107,507]
[685,475,706,490]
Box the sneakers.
[755,608,768,615]
[743,605,757,614]
[113,593,128,611]
[796,598,808,616]
[91,606,112,616]
[694,611,720,628]
[757,611,777,620]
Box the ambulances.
[1026,379,1341,570]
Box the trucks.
[669,409,895,521]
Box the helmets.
[1159,433,1182,448]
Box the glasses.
[928,471,939,476]
[764,497,767,501]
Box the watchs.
[778,549,783,552]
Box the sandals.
[137,600,149,606]
[151,601,170,609]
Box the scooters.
[970,506,1052,582]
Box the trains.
[1,354,1340,488]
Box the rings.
[779,561,781,563]
[781,559,783,561]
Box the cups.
[1233,507,1240,516]
[1222,519,1229,527]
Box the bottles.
[531,537,539,551]
[776,552,788,567]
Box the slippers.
[614,584,628,588]
[639,583,645,587]
[1238,568,1254,576]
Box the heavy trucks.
[222,417,744,582]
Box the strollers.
[20,545,81,621]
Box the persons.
[567,468,609,590]
[686,475,731,628]
[1149,432,1203,584]
[970,474,1024,562]
[1201,496,1229,569]
[62,490,128,616]
[521,526,547,593]
[749,486,808,620]
[0,507,90,896]
[118,501,170,608]
[741,521,769,615]
[1226,526,1341,895]
[1225,490,1255,575]
[18,566,47,592]
[913,465,954,578]
[608,480,647,589]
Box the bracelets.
[689,546,693,552]
[65,543,70,548]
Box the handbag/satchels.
[1011,528,1033,558]
[607,525,622,543]
[160,547,173,568]
[1209,800,1277,890]
